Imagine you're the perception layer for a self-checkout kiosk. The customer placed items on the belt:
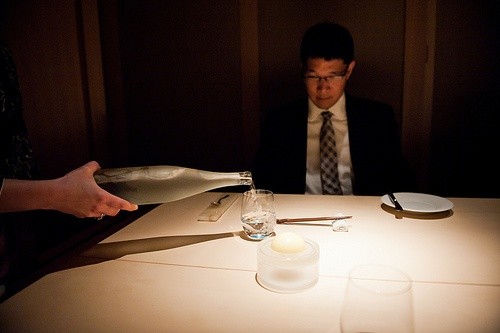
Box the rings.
[96,213,103,220]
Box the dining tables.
[0,192,500,333]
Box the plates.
[380,192,453,213]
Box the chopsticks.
[277,215,353,224]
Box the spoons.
[211,194,230,205]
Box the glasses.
[304,65,348,83]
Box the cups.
[240,190,276,241]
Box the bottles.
[92,166,253,206]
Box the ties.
[319,111,343,195]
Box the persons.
[249,23,400,196]
[0,160,138,219]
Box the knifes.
[388,192,403,210]
